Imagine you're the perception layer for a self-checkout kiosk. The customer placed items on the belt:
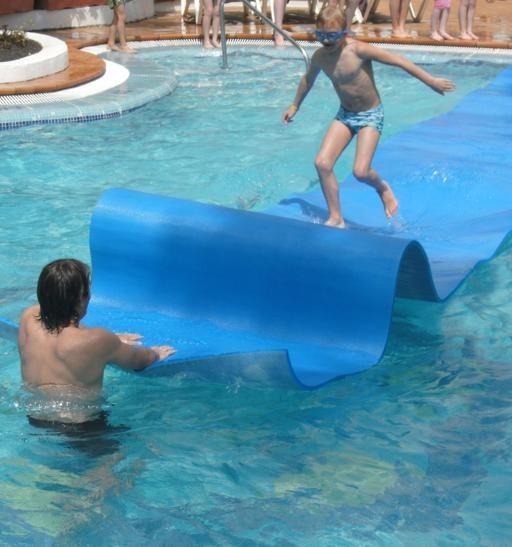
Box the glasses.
[315,28,347,41]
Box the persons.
[273,0,291,47]
[202,0,222,49]
[329,0,360,37]
[388,0,413,38]
[430,0,454,41]
[18,259,175,456]
[282,8,458,230]
[459,1,480,41]
[107,0,137,53]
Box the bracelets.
[290,103,299,112]
